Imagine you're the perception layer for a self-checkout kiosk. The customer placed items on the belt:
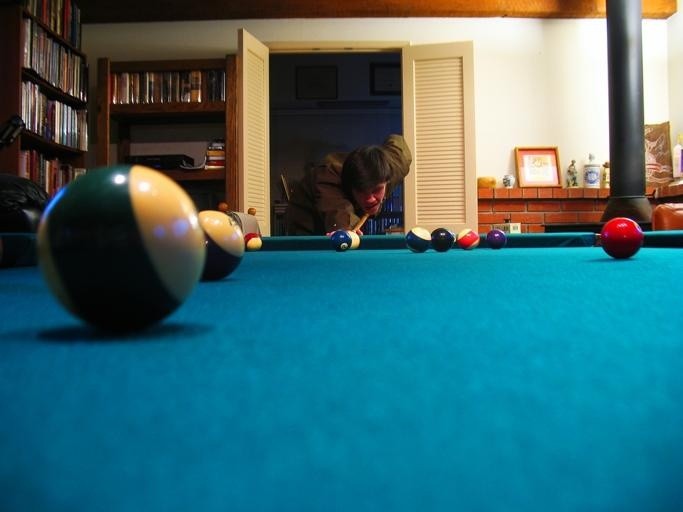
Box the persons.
[281,132,411,240]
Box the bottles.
[583,152,610,188]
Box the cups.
[501,173,517,188]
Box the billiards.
[330,229,361,251]
[35,165,206,336]
[197,210,245,282]
[405,228,506,252]
[243,233,262,250]
[599,217,643,258]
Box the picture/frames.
[514,145,564,189]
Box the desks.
[0,220,682,511]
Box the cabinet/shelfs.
[0,0,89,199]
[97,53,236,208]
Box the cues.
[351,213,370,232]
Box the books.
[110,69,226,103]
[16,0,90,197]
